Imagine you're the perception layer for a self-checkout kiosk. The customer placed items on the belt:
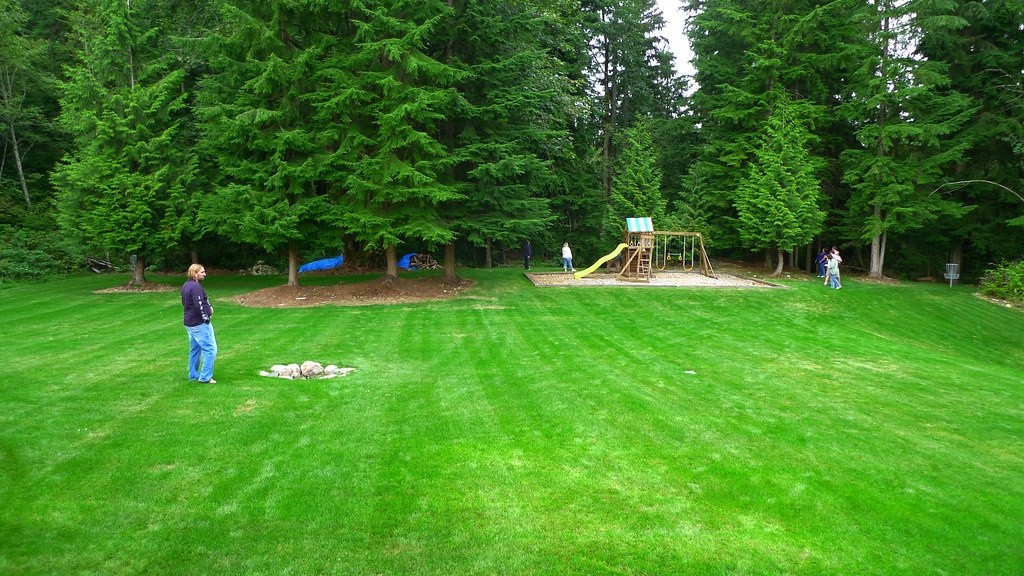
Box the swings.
[656,234,666,271]
[683,236,694,272]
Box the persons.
[181,263,217,385]
[561,241,577,272]
[523,240,532,270]
[814,246,843,290]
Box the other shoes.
[817,275,825,277]
[824,282,827,286]
[571,268,576,271]
[208,379,217,384]
[836,285,841,289]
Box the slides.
[572,242,628,279]
